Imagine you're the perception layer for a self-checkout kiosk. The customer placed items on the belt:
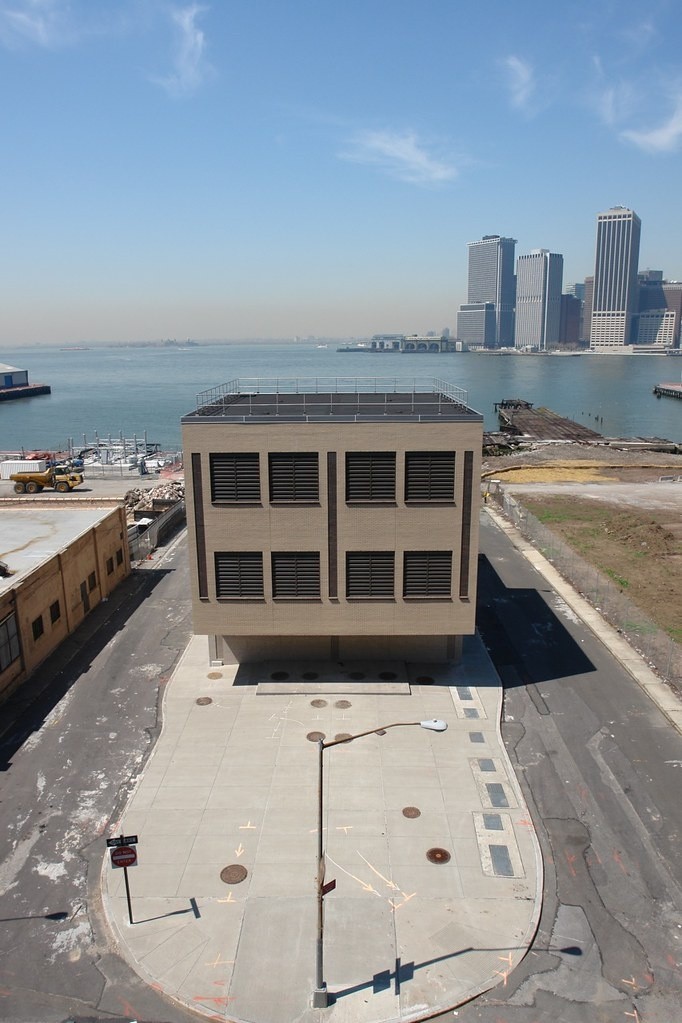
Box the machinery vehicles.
[9,465,84,494]
